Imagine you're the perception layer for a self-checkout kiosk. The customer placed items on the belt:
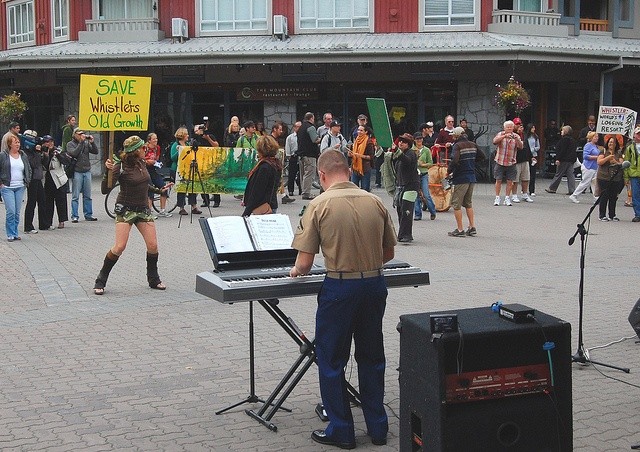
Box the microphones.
[611,160,631,181]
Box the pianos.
[195,215,430,431]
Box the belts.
[325,270,383,280]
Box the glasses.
[141,146,146,150]
[448,121,454,123]
[399,138,409,143]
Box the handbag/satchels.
[252,162,277,214]
[113,203,148,215]
[61,142,78,178]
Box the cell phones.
[430,314,458,333]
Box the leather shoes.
[150,281,167,290]
[311,429,356,449]
[414,216,422,221]
[400,237,412,242]
[201,203,209,207]
[94,278,104,295]
[213,203,219,208]
[58,222,64,229]
[431,214,436,220]
[282,195,295,204]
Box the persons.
[170,127,202,215]
[285,121,303,196]
[353,114,373,138]
[436,115,457,143]
[193,124,220,207]
[297,111,321,199]
[525,124,540,196]
[224,115,241,147]
[145,132,173,219]
[460,119,475,141]
[43,135,71,230]
[413,132,435,220]
[545,125,577,195]
[414,123,429,149]
[448,127,477,237]
[1,122,20,153]
[320,120,347,153]
[352,126,374,192]
[94,135,166,294]
[275,120,290,147]
[511,118,534,203]
[570,131,600,203]
[394,133,416,245]
[226,123,239,147]
[290,149,395,449]
[624,127,640,222]
[256,121,268,136]
[598,138,625,221]
[22,129,55,234]
[67,127,98,223]
[578,115,597,145]
[317,113,333,144]
[544,119,557,142]
[426,121,437,147]
[61,115,76,151]
[604,134,624,147]
[270,123,295,204]
[236,120,259,148]
[493,120,524,206]
[0,135,32,242]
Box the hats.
[43,135,53,143]
[419,123,431,129]
[513,117,522,125]
[330,120,342,126]
[400,133,413,142]
[123,136,144,153]
[634,127,640,134]
[244,121,255,127]
[358,114,366,120]
[453,127,465,135]
[414,132,423,139]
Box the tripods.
[568,174,622,372]
[198,214,298,414]
[178,162,213,228]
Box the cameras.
[509,134,515,140]
[16,133,49,154]
[84,134,91,141]
[198,116,209,136]
[182,142,198,160]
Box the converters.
[498,303,535,323]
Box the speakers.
[400,302,573,451]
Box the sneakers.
[545,188,556,193]
[613,217,619,222]
[24,230,38,234]
[48,226,56,231]
[192,208,202,214]
[531,193,536,197]
[158,211,173,218]
[465,227,477,235]
[503,196,513,206]
[598,217,612,222]
[632,217,639,222]
[86,217,98,221]
[179,209,188,215]
[151,208,159,220]
[511,194,520,203]
[72,218,78,223]
[18,236,22,240]
[8,236,14,242]
[448,229,466,237]
[521,193,534,203]
[494,196,501,206]
[569,194,580,203]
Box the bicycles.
[105,172,180,220]
